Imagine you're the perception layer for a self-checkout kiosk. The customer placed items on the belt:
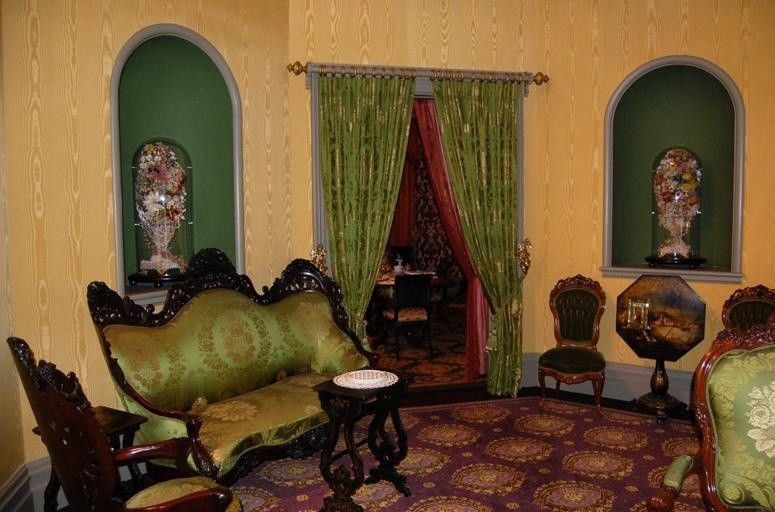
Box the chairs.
[431,257,453,330]
[537,275,608,417]
[645,283,774,511]
[383,271,434,362]
[6,336,243,512]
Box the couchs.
[87,247,383,488]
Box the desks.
[311,367,417,512]
[376,269,457,289]
[32,406,148,512]
